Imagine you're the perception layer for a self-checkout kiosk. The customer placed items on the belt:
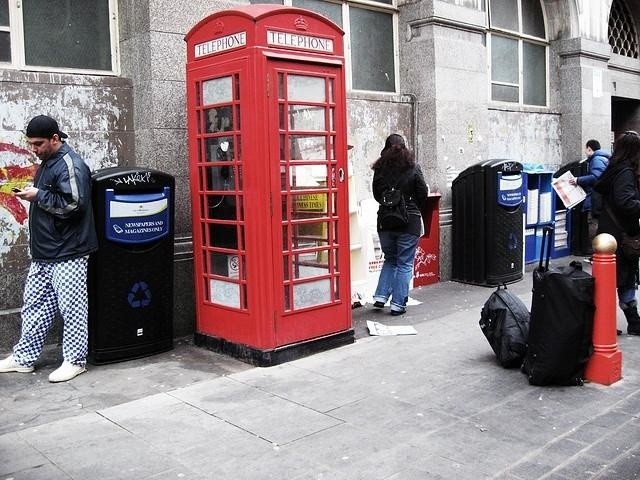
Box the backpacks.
[479,281,530,369]
[380,184,409,230]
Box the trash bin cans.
[413,193,441,288]
[451,159,523,288]
[86,166,175,366]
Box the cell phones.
[11,186,24,192]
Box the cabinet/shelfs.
[523,171,572,264]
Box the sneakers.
[49,360,85,383]
[0,354,35,372]
[373,302,384,308]
[391,310,406,315]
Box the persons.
[590,131,640,337]
[1,115,98,383]
[568,139,612,263]
[371,134,429,316]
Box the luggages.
[521,225,594,386]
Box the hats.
[26,115,68,138]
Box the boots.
[619,300,640,335]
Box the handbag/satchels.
[619,232,640,259]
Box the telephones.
[207,143,243,207]
